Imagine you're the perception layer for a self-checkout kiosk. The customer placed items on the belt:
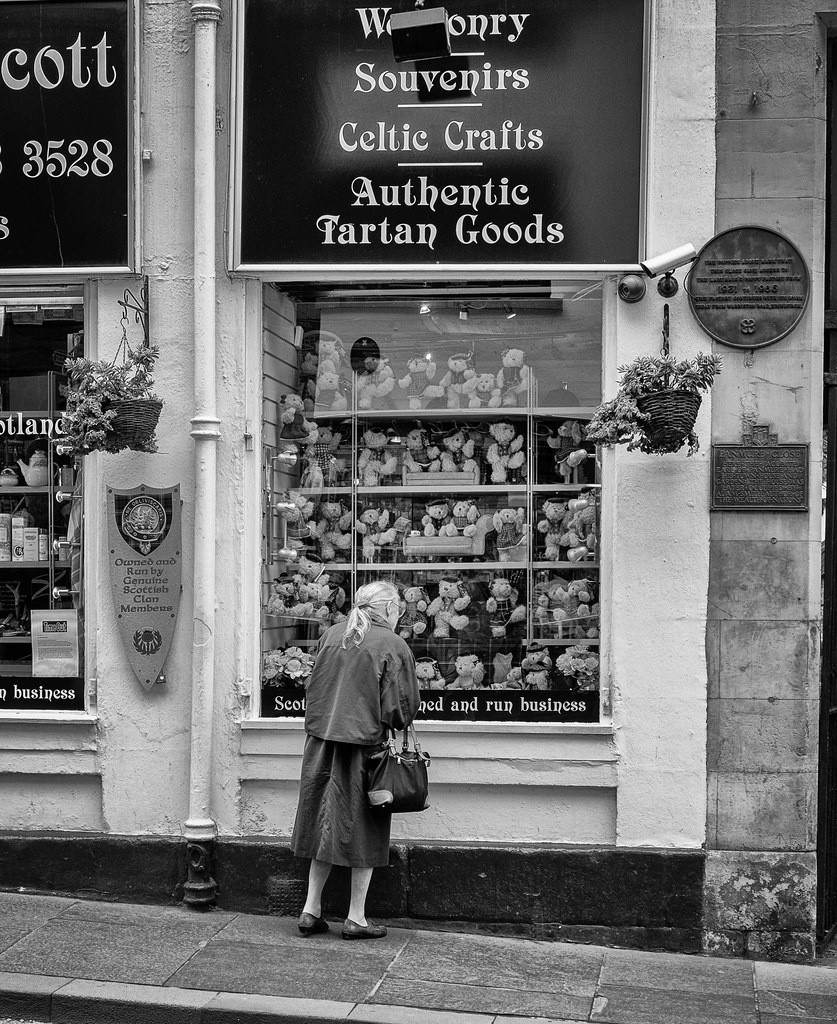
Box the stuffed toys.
[277,393,317,451]
[490,506,528,562]
[357,429,398,487]
[426,576,470,639]
[311,501,353,559]
[355,505,396,558]
[259,339,598,694]
[486,422,523,481]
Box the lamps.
[639,243,697,299]
[502,302,519,321]
[387,0,452,63]
[456,306,471,322]
[419,300,433,315]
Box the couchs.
[403,514,495,562]
[402,466,480,484]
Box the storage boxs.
[0,514,48,562]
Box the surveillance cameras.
[639,243,697,279]
[618,275,646,303]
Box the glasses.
[384,602,405,618]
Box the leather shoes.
[342,917,387,940]
[298,912,329,935]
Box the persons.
[289,579,421,941]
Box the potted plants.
[50,340,169,457]
[586,349,723,458]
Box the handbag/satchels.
[367,720,431,813]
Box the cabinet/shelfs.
[267,366,599,647]
[0,411,78,678]
